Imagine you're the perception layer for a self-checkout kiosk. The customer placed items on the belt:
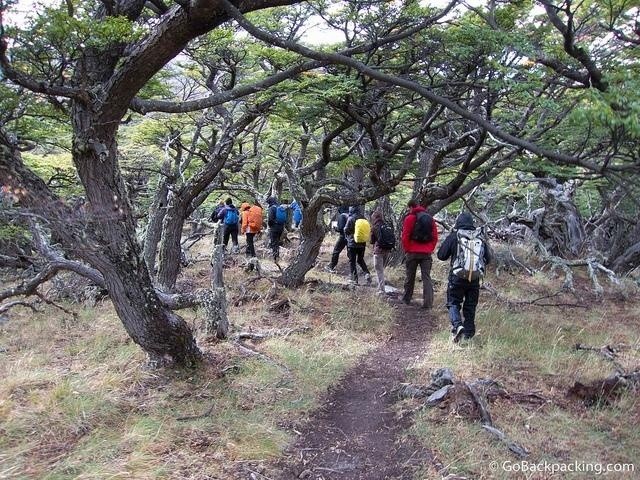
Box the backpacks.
[453,227,486,282]
[248,205,262,230]
[411,212,433,241]
[354,218,370,243]
[225,210,239,225]
[275,206,288,223]
[377,223,396,250]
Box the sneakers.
[348,279,358,285]
[452,326,465,343]
[418,306,432,311]
[398,295,409,304]
[324,265,335,272]
[365,273,372,285]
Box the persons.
[212,200,225,246]
[397,198,439,308]
[288,196,302,228]
[323,205,349,272]
[266,195,284,261]
[239,201,258,256]
[437,212,491,346]
[214,198,239,254]
[343,205,372,287]
[369,210,391,293]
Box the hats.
[241,202,250,210]
[226,198,232,205]
